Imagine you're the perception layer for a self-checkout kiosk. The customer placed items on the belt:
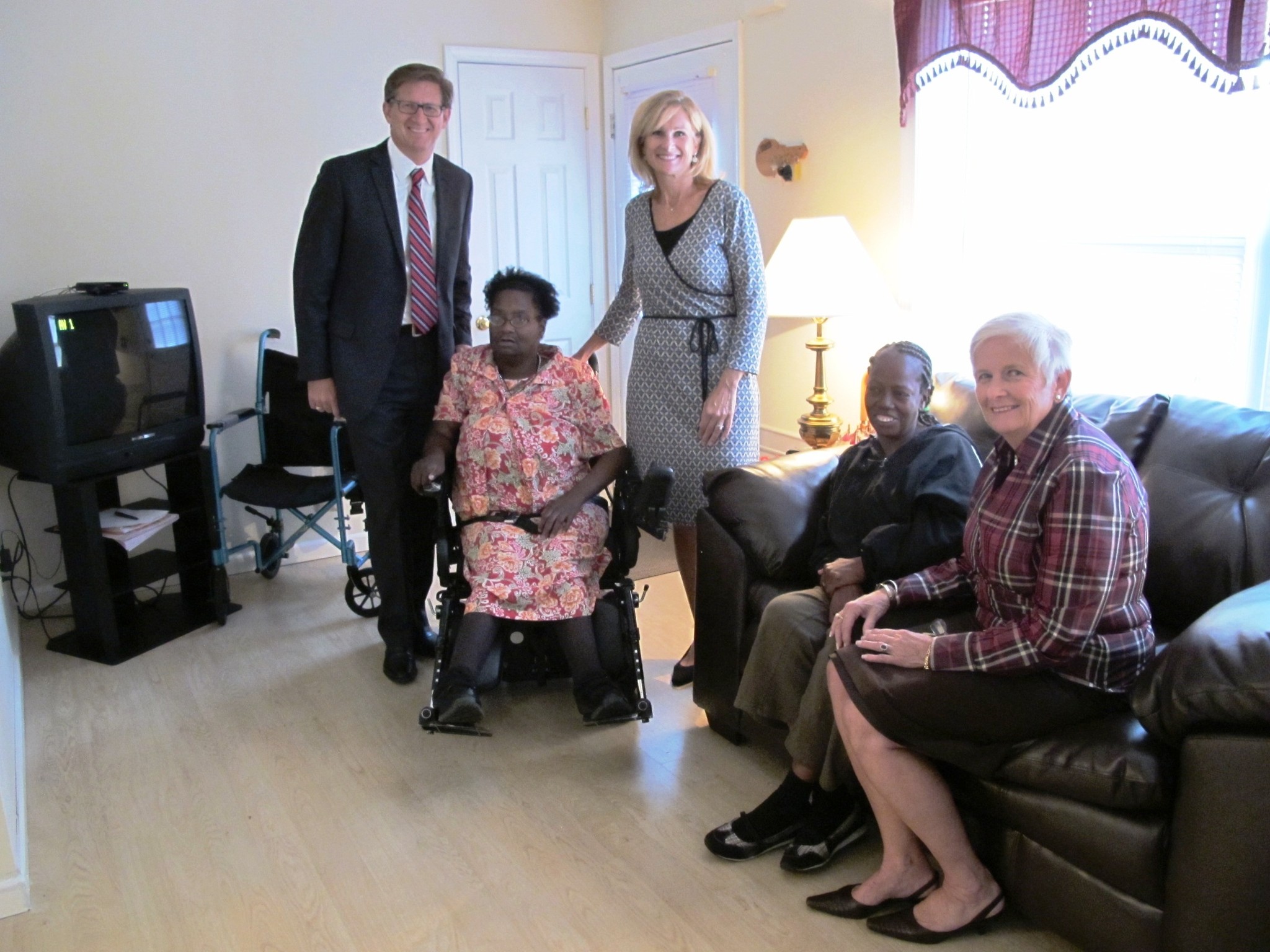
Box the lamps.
[762,213,881,449]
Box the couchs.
[690,388,1270,952]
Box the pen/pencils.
[115,510,138,520]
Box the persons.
[576,91,767,687]
[705,343,987,874]
[410,267,631,730]
[292,64,473,687]
[807,314,1154,945]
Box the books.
[98,507,180,550]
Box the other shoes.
[780,804,873,874]
[867,887,1008,944]
[806,873,940,921]
[436,665,484,723]
[671,645,694,687]
[575,673,633,727]
[704,799,811,862]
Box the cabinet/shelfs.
[14,444,242,657]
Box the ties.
[408,168,439,333]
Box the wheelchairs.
[417,352,677,738]
[206,329,384,626]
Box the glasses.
[389,99,447,117]
[487,313,544,327]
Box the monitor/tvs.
[0,286,205,483]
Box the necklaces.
[495,352,541,392]
[670,208,674,211]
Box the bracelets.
[876,579,899,608]
[924,641,930,669]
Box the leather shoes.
[407,628,439,659]
[383,642,417,684]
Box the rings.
[821,581,825,586]
[315,406,323,410]
[880,643,888,653]
[835,613,844,620]
[716,424,724,430]
